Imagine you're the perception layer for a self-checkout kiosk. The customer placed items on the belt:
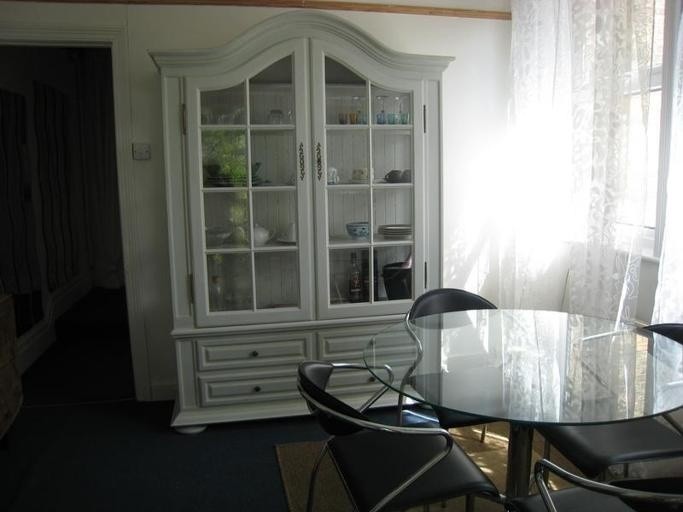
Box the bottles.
[210,275,222,310]
[346,252,363,303]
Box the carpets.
[275,414,588,512]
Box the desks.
[362,307,683,500]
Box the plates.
[378,224,412,240]
[203,175,261,187]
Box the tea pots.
[237,224,274,246]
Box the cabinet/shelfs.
[148,9,457,436]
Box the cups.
[385,169,411,183]
[339,112,409,124]
[327,166,339,184]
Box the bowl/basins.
[346,222,370,240]
[204,163,260,176]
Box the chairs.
[396,288,533,441]
[534,322,683,485]
[299,356,503,510]
[533,455,682,511]
